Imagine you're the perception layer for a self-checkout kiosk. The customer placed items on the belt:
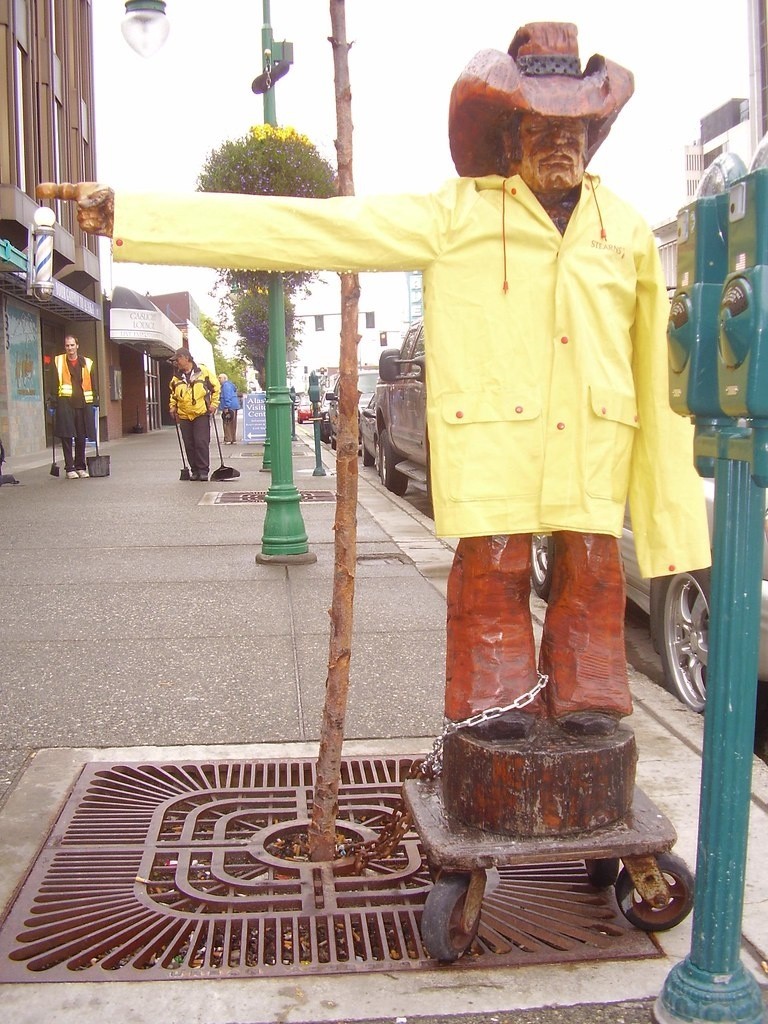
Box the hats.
[169,347,189,361]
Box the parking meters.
[290,386,298,442]
[648,132,768,1024]
[308,368,326,477]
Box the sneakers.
[79,469,90,479]
[65,469,78,479]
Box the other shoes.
[222,441,236,445]
[199,471,209,482]
[188,468,199,480]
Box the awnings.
[109,285,183,362]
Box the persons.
[218,373,239,444]
[168,346,220,481]
[39,23,712,740]
[47,334,101,479]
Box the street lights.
[115,1,316,567]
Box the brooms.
[173,410,192,481]
[48,398,60,478]
[210,412,240,482]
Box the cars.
[294,390,334,443]
[527,477,768,716]
[361,392,380,472]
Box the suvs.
[324,368,381,450]
[373,316,426,493]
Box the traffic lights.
[380,332,387,347]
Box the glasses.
[171,356,184,364]
